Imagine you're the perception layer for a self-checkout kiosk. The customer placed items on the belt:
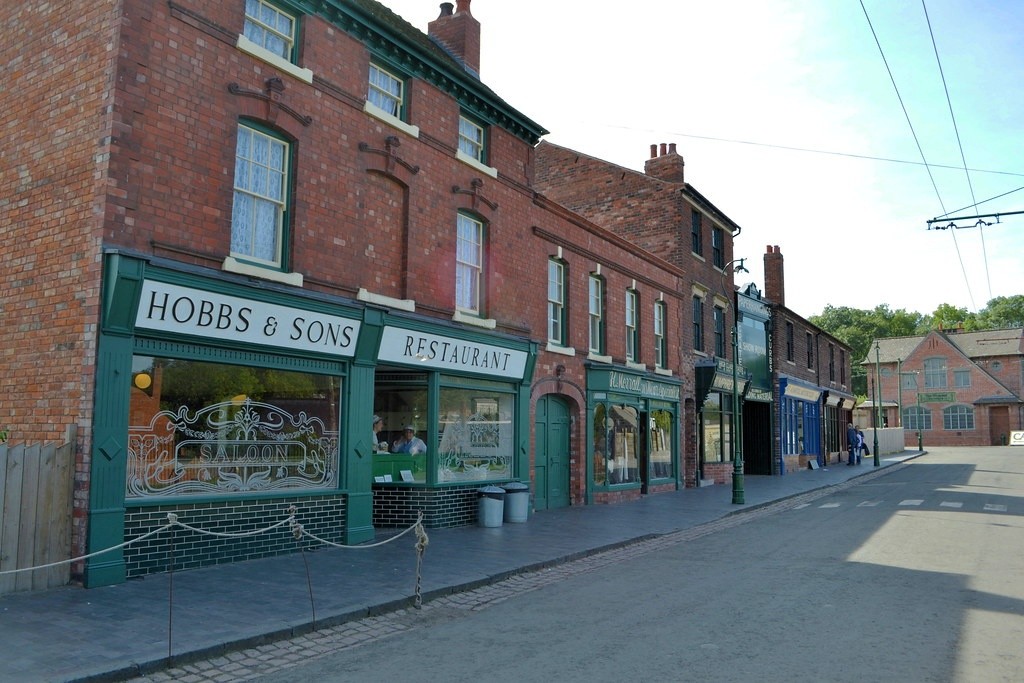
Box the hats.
[373,415,381,424]
[405,425,415,431]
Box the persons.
[373,415,383,452]
[846,424,864,466]
[392,424,427,456]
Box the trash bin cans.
[476,486,506,527]
[500,482,530,523]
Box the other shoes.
[847,463,854,466]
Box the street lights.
[896,356,925,452]
[860,339,884,468]
[721,257,752,505]
